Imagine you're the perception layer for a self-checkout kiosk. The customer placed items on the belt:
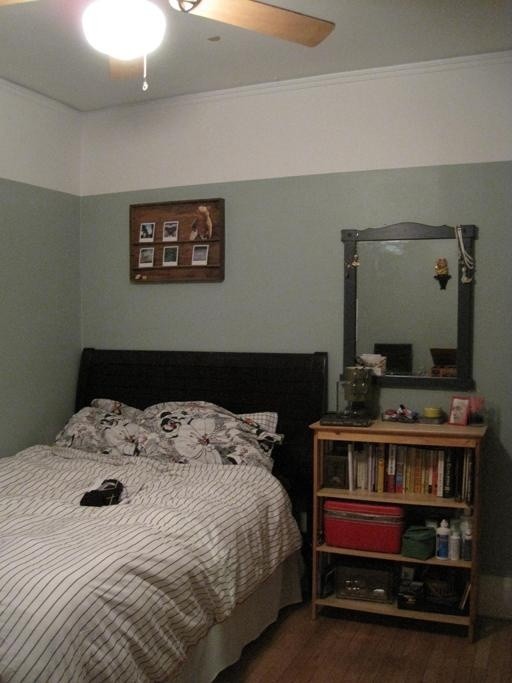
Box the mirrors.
[340,221,477,392]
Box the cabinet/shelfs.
[308,416,488,644]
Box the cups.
[352,381,368,420]
[415,349,427,376]
[336,381,352,419]
[428,357,441,376]
[425,408,444,418]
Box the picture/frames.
[447,396,471,426]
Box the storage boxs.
[323,499,407,554]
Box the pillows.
[55,399,143,456]
[138,401,285,464]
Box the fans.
[0,0,336,48]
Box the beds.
[1,348,329,683]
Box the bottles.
[435,519,474,561]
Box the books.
[344,441,460,499]
